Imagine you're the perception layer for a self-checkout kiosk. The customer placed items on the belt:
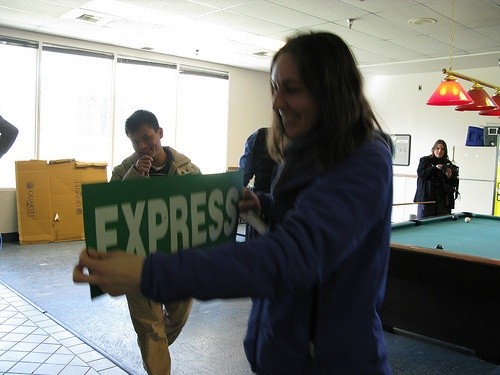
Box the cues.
[392,200,436,207]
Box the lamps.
[427,0,500,116]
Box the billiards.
[435,243,444,250]
[451,215,458,221]
[464,216,471,223]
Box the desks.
[383,212,500,363]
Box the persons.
[74,32,394,375]
[413,140,458,219]
[108,110,201,375]
[237,126,279,241]
